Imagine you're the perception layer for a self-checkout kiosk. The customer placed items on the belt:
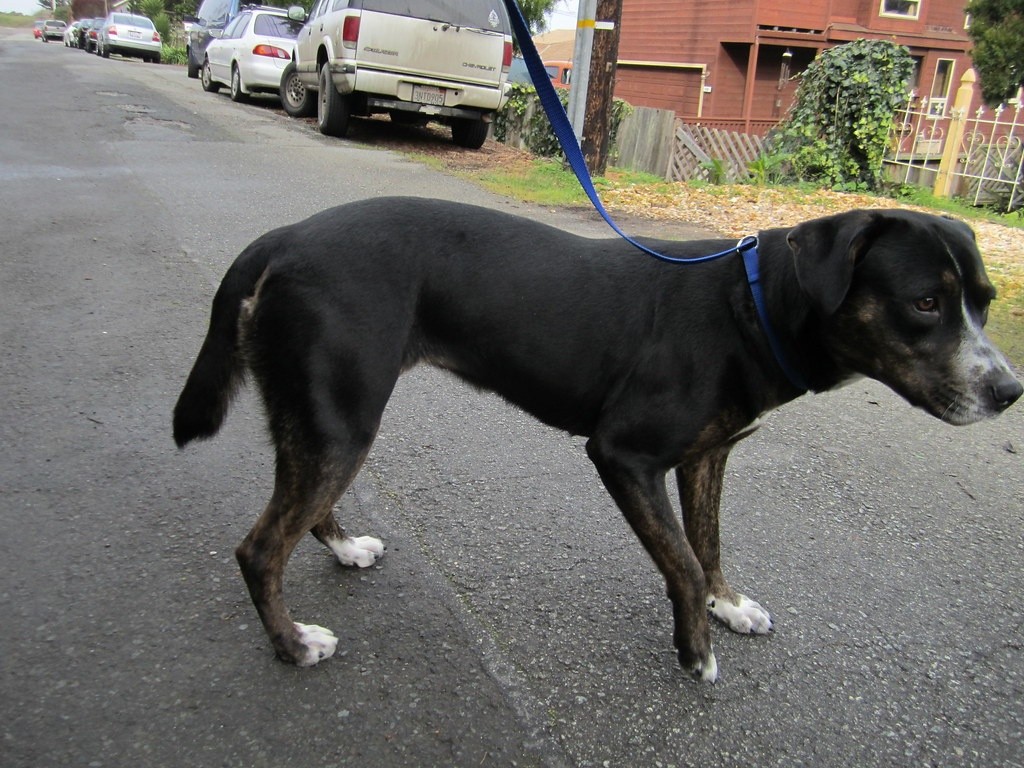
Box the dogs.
[173,194,1023,687]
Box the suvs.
[277,0,515,151]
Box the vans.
[184,0,270,77]
[537,59,572,93]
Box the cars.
[32,16,107,53]
[94,12,163,64]
[200,3,310,104]
